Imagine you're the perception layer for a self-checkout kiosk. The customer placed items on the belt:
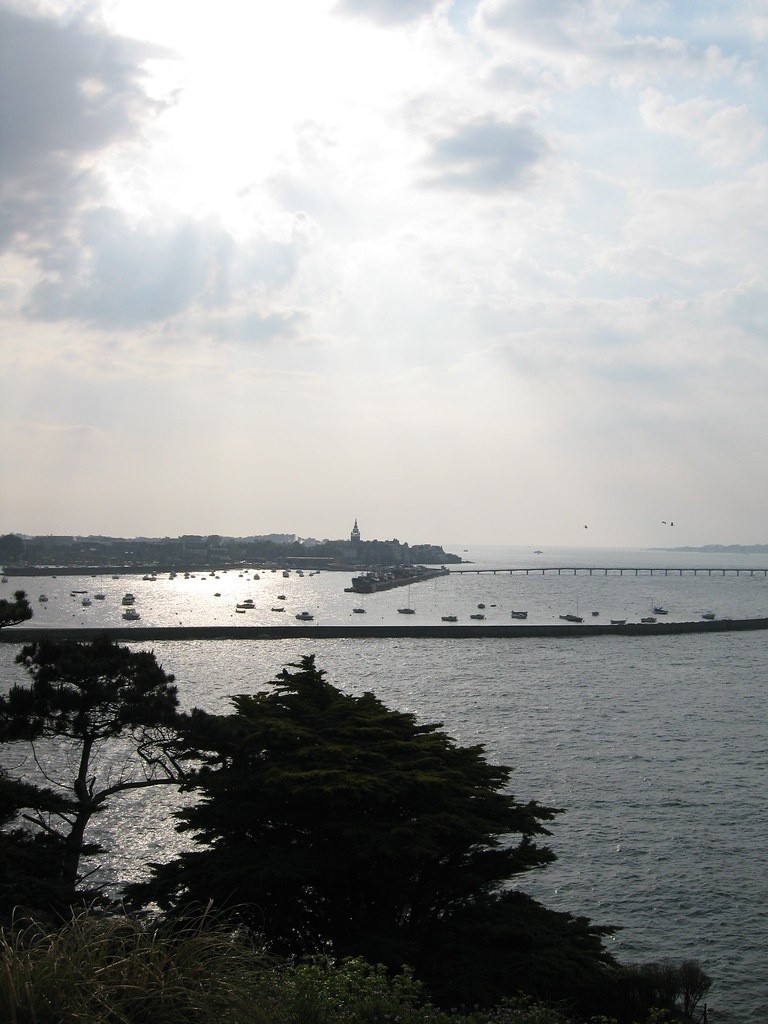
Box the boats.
[295,611,315,620]
[90,568,321,581]
[478,603,485,609]
[235,608,246,613]
[353,608,367,614]
[559,607,715,624]
[38,594,48,602]
[397,608,416,614]
[470,613,485,619]
[94,594,105,599]
[271,607,286,613]
[121,597,134,605]
[441,615,459,622]
[125,592,136,602]
[122,607,141,620]
[71,586,88,593]
[511,610,527,619]
[81,597,92,607]
[214,593,221,596]
[277,595,286,600]
